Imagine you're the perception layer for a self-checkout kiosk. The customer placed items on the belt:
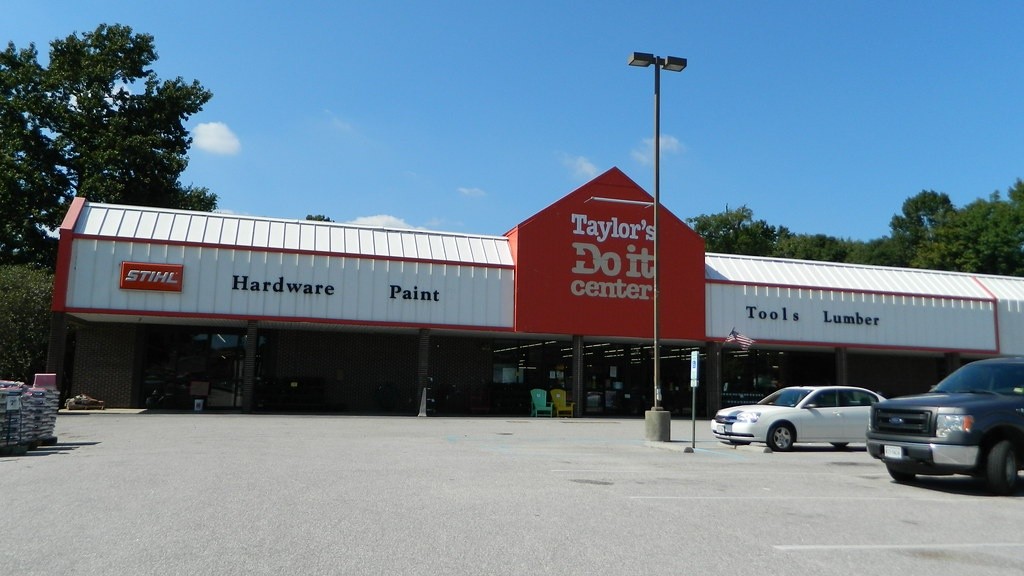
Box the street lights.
[627,52,687,443]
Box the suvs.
[865,354,1024,495]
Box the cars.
[709,385,885,452]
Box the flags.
[721,326,756,351]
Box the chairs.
[550,389,576,417]
[530,389,554,418]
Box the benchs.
[849,401,876,405]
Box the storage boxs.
[35,374,56,386]
